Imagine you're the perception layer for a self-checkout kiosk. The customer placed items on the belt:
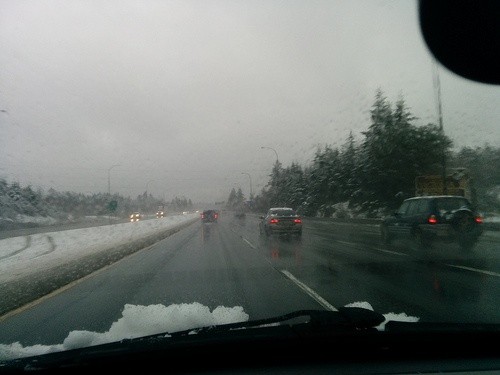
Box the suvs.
[379,195,485,253]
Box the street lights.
[261,146,279,172]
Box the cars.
[156,211,165,216]
[259,207,303,240]
[130,211,142,219]
[200,209,217,224]
